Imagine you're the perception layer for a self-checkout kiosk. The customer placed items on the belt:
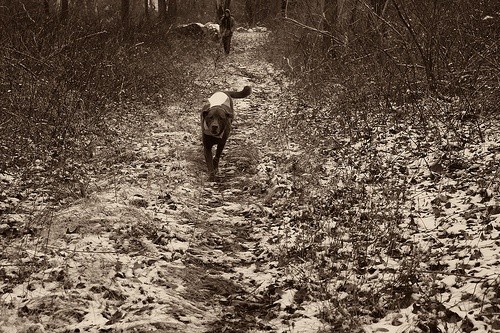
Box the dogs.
[200,86,251,182]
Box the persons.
[219,9,236,56]
